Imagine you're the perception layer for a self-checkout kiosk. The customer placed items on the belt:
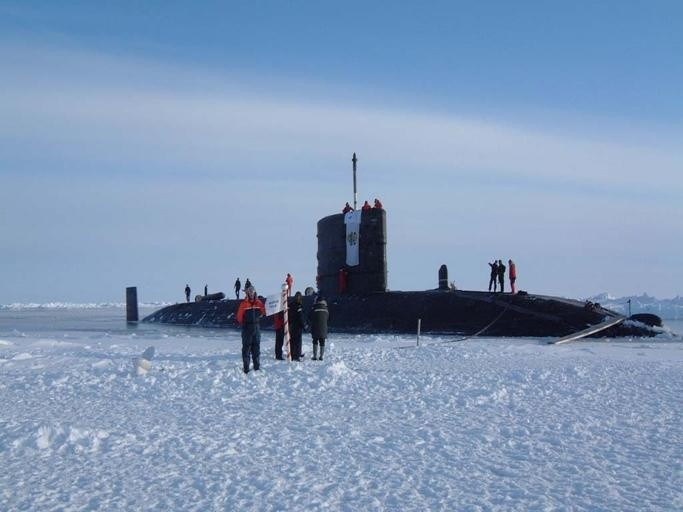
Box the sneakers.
[243,366,259,372]
[275,354,303,362]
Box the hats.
[245,287,256,301]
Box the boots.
[311,344,324,360]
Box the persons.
[233,277,240,300]
[508,258,517,295]
[204,284,208,297]
[243,278,251,300]
[274,306,291,361]
[308,294,331,361]
[185,283,191,303]
[337,266,348,295]
[496,259,507,293]
[236,285,266,375]
[287,290,306,362]
[342,201,354,214]
[361,200,372,210]
[373,197,382,209]
[285,273,293,296]
[488,259,499,292]
